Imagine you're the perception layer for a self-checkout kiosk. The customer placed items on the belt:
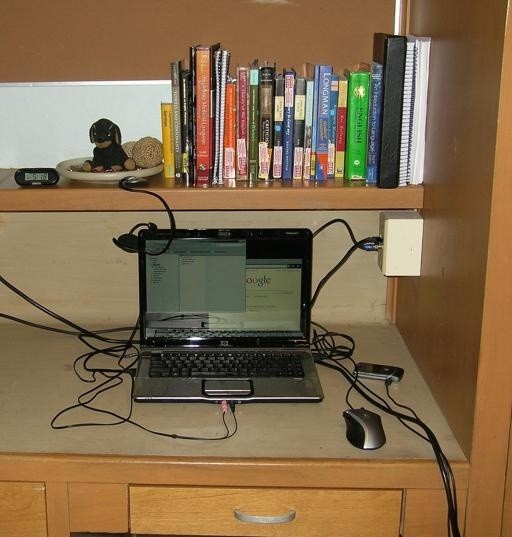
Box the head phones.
[112,177,175,256]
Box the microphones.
[128,222,158,236]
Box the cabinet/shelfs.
[0,0,512,536]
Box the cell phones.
[353,362,405,383]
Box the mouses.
[343,408,386,450]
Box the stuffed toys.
[81,117,137,170]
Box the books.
[162,31,432,188]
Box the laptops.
[134,229,324,403]
